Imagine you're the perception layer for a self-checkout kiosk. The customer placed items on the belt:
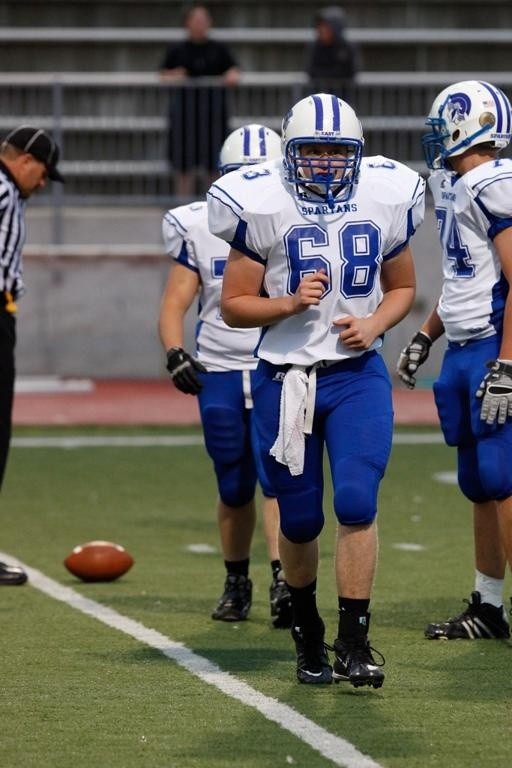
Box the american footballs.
[63,540,135,582]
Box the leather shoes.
[0,563,25,584]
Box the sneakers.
[332,639,385,688]
[291,617,333,684]
[270,581,292,616]
[211,580,253,621]
[424,591,510,640]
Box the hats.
[4,125,65,183]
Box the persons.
[394,80,512,645]
[303,5,357,110]
[0,127,63,588]
[159,121,293,631]
[158,7,242,199]
[207,93,430,689]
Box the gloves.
[476,358,512,428]
[397,331,432,390]
[166,347,207,395]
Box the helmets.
[421,80,512,169]
[281,93,365,204]
[217,124,281,177]
